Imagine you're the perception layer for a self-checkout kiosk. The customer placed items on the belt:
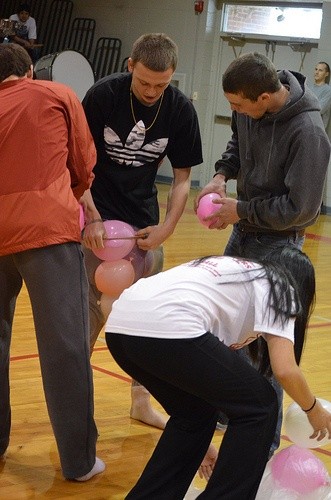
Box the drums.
[33,49,95,105]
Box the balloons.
[285,398,331,448]
[272,446,328,495]
[196,192,223,227]
[79,203,154,319]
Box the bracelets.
[301,397,316,412]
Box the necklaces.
[130,87,164,131]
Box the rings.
[322,432,327,435]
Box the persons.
[80,32,203,430]
[193,52,331,428]
[0,43,105,481]
[8,7,37,63]
[308,61,331,130]
[105,240,331,500]
